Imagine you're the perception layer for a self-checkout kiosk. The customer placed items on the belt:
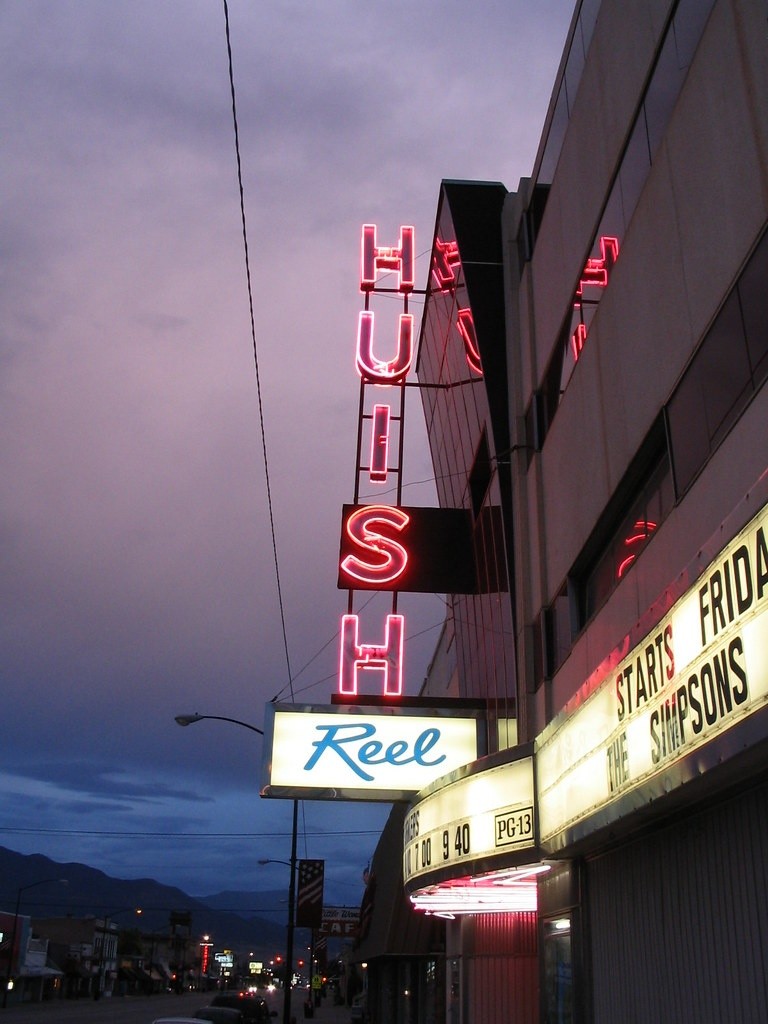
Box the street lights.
[99,907,143,959]
[256,858,313,999]
[2,878,69,1008]
[173,712,298,1024]
[181,933,209,986]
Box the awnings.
[22,962,65,976]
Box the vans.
[212,990,279,1024]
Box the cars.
[151,1017,213,1024]
[193,1005,257,1024]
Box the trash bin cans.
[350,1004,365,1024]
